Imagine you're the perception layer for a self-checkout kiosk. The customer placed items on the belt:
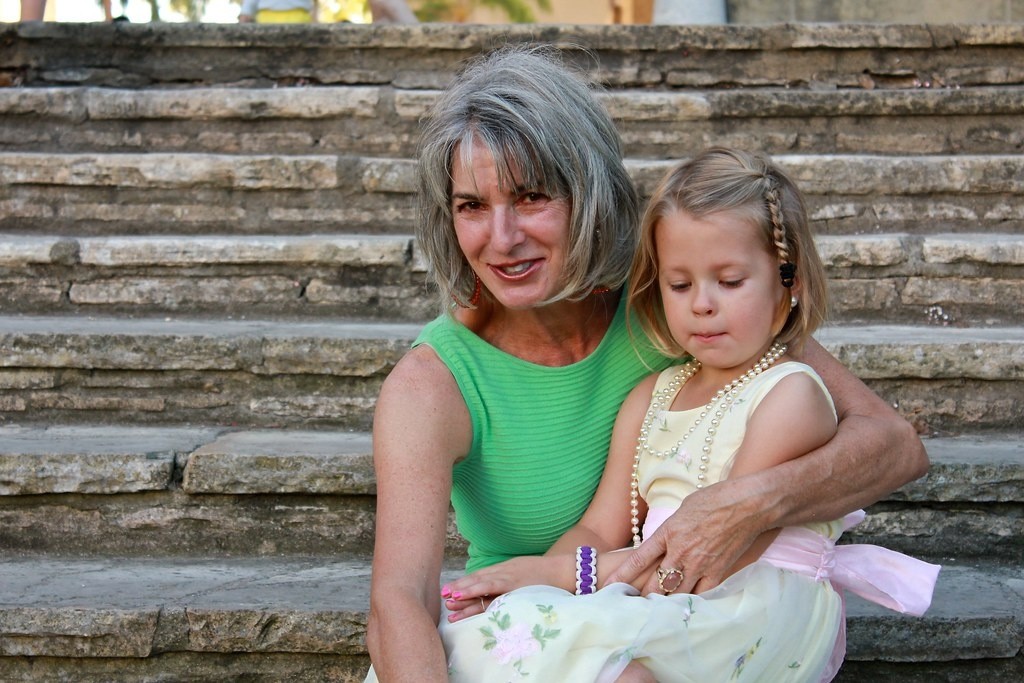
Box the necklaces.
[630,339,788,550]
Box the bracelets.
[576,546,597,595]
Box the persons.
[235,0,420,27]
[360,144,852,683]
[366,52,930,683]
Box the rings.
[656,565,684,594]
[480,594,493,612]
